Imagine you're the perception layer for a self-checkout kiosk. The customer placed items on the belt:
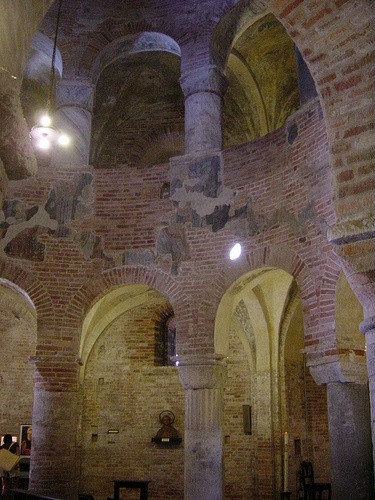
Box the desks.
[113,479,151,500]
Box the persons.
[154,415,181,438]
[0,433,21,500]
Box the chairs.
[300,461,332,499]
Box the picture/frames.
[18,423,33,456]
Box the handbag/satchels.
[0,442,21,472]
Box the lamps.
[28,1,75,151]
